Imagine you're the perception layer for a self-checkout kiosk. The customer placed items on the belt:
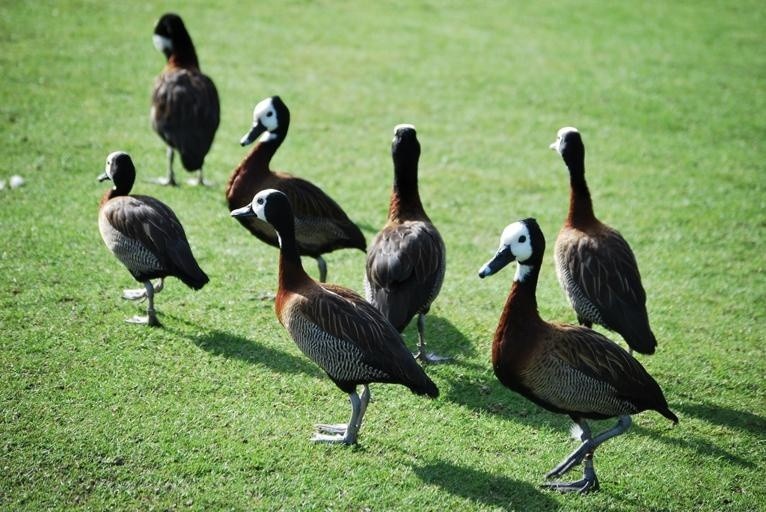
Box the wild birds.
[362,122,452,364]
[477,216,677,497]
[93,149,212,327]
[223,94,368,284]
[141,12,221,190]
[548,125,658,358]
[229,186,440,446]
